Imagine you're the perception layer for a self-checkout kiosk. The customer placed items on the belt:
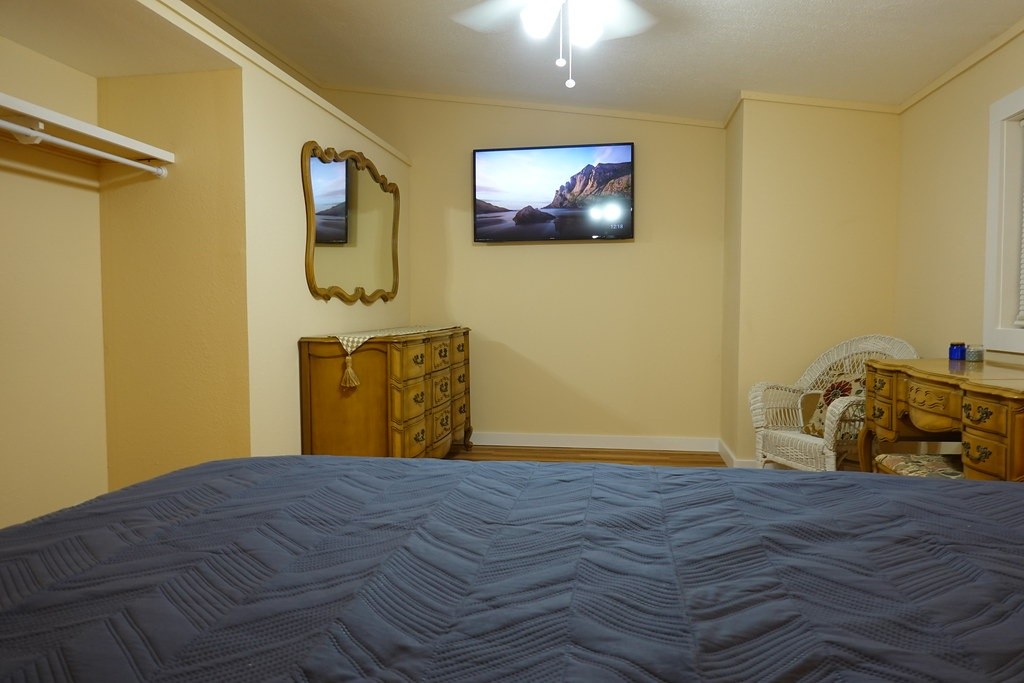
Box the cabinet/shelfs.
[299,325,472,459]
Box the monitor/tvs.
[473,142,635,243]
[310,156,348,243]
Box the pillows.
[802,372,867,438]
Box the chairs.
[745,331,920,473]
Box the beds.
[0,458,1024,683]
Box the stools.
[872,453,963,479]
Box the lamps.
[519,0,600,89]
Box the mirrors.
[300,140,402,303]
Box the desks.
[857,357,1024,483]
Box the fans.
[446,0,658,43]
